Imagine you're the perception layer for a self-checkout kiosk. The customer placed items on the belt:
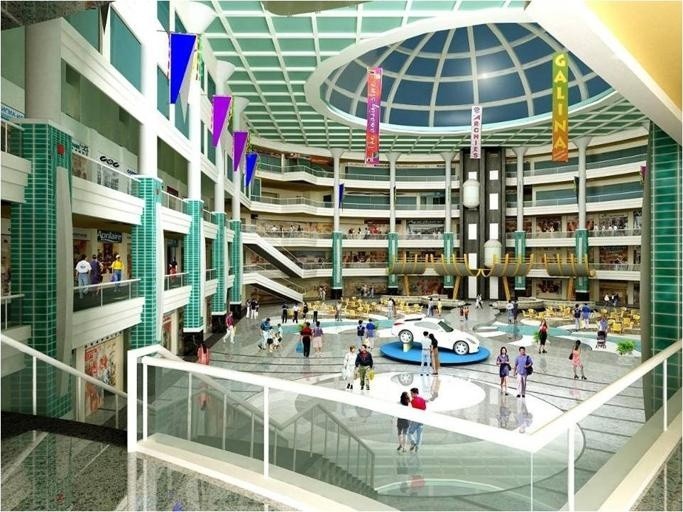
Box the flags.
[169,30,199,106]
[231,131,249,171]
[211,93,231,145]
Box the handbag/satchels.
[569,346,574,360]
[296,339,303,352]
[507,356,511,370]
[526,356,533,375]
[369,368,375,380]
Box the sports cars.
[392,314,481,355]
[391,372,423,392]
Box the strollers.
[593,329,608,349]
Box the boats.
[490,296,546,312]
[421,295,467,309]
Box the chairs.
[518,300,640,334]
[280,296,424,321]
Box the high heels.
[582,375,587,379]
[574,375,579,378]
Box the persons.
[421,331,439,375]
[397,388,426,452]
[345,344,373,390]
[407,279,483,321]
[73,157,179,300]
[223,285,395,358]
[252,146,324,176]
[506,211,641,271]
[396,454,412,497]
[497,346,533,398]
[573,339,587,380]
[406,451,425,500]
[252,223,442,270]
[506,282,620,354]
[197,342,208,364]
